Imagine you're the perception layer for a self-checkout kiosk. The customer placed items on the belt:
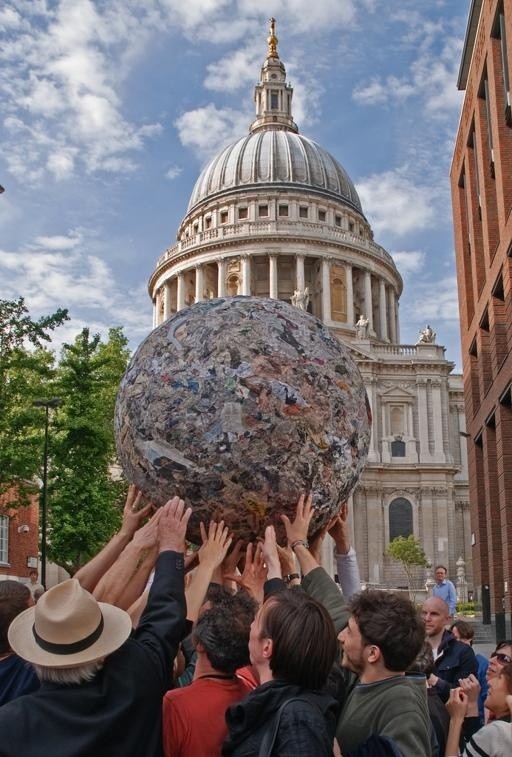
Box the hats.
[6,576,134,668]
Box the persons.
[0,485,511,757]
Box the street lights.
[29,397,65,589]
